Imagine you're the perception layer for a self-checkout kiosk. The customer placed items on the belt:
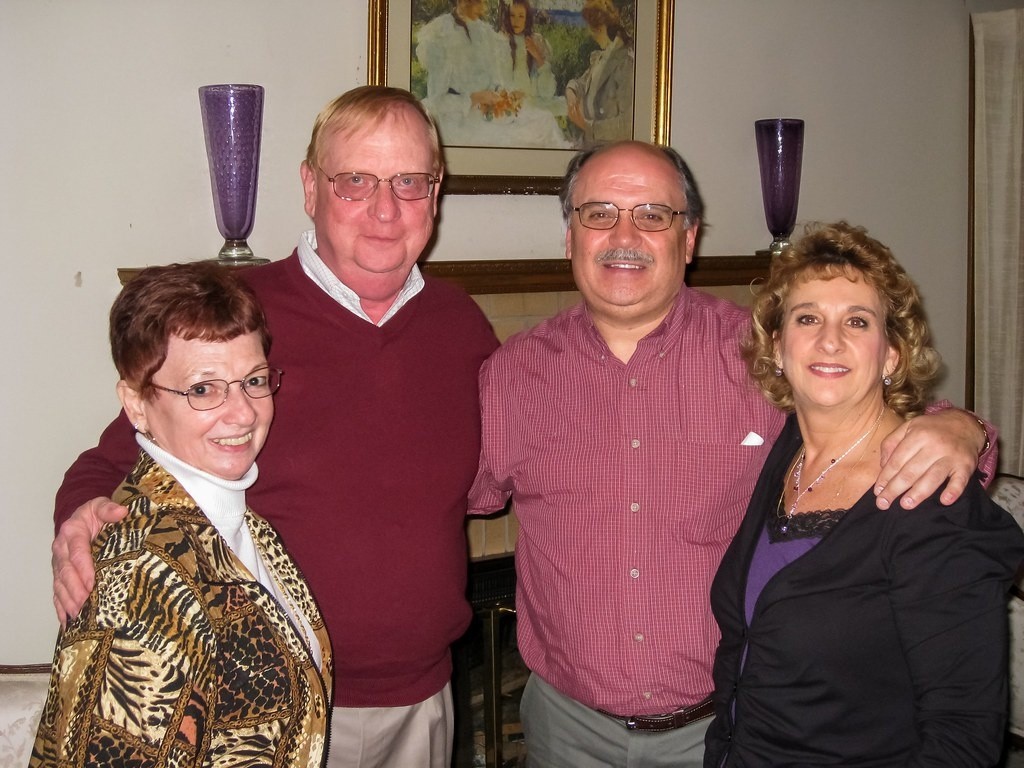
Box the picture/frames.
[366,0,677,196]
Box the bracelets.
[958,409,991,456]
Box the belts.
[597,697,713,734]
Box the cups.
[198,83,270,266]
[755,117,805,256]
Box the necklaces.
[777,405,885,531]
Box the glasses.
[317,165,440,201]
[570,202,687,232]
[148,365,283,411]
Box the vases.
[754,117,805,267]
[198,83,272,266]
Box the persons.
[415,0,575,150]
[51,87,500,768]
[468,138,1000,768]
[495,0,558,104]
[567,0,635,151]
[28,262,337,768]
[701,214,1024,767]
